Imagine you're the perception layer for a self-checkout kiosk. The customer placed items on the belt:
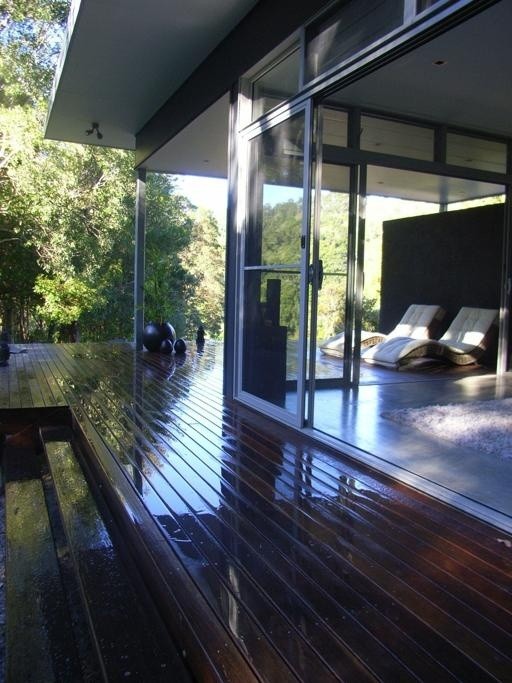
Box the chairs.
[319,302,445,360]
[362,306,499,369]
